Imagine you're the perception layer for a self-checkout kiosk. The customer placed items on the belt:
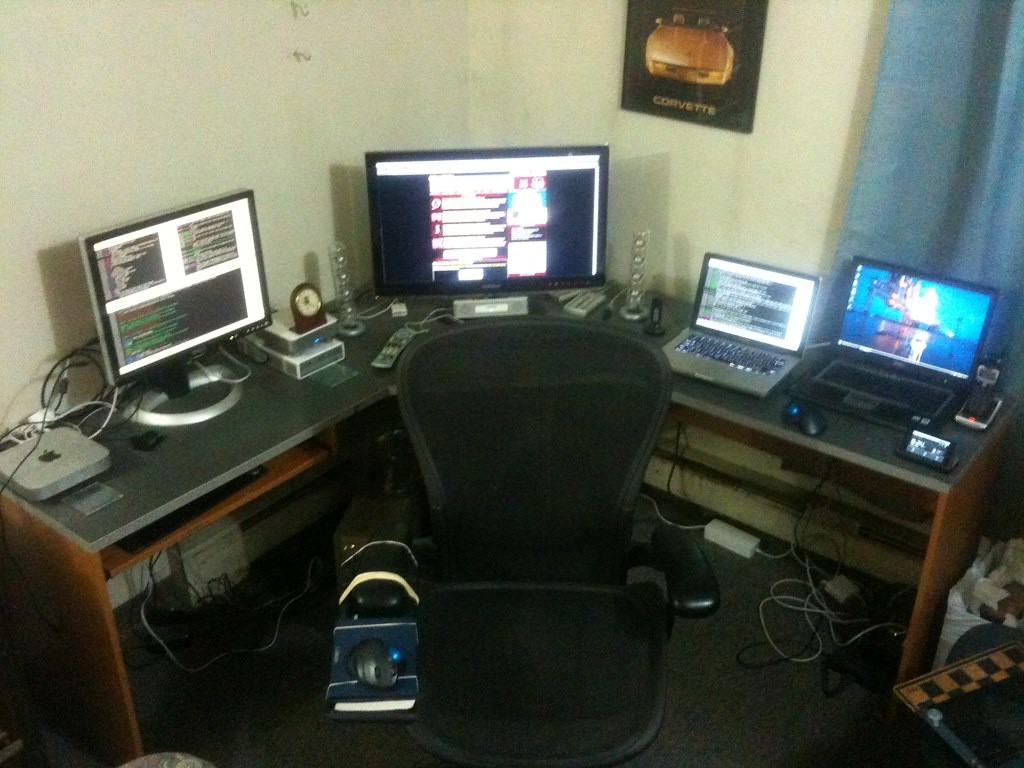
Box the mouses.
[783,397,827,438]
[346,636,401,693]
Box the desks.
[0,277,1019,765]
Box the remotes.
[370,327,417,369]
[562,291,606,317]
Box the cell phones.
[953,393,1002,431]
[899,426,957,470]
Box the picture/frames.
[622,0,770,135]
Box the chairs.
[351,313,722,768]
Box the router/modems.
[0,425,114,505]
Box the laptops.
[784,253,1002,440]
[661,251,824,402]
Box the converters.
[703,518,760,561]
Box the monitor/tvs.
[363,143,610,296]
[79,187,274,388]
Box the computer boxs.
[334,488,419,596]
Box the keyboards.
[114,464,270,554]
